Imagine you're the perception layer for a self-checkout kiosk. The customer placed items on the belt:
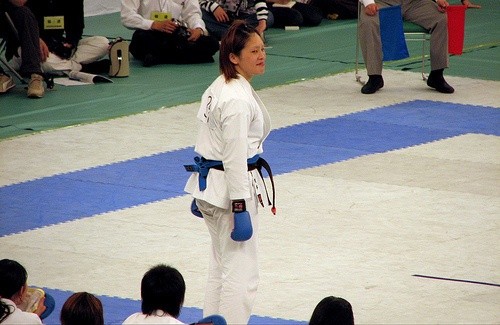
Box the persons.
[308,296,354,325]
[0,258,43,325]
[184,20,276,325]
[0,0,362,97]
[122,265,186,325]
[60,291,104,325]
[359,0,455,94]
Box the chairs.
[355,2,430,84]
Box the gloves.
[191,198,203,218]
[231,211,253,242]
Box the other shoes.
[79,59,111,75]
[42,72,54,89]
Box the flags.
[445,5,468,55]
[379,5,409,61]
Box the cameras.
[178,26,190,38]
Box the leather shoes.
[361,74,384,94]
[427,70,455,94]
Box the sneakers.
[0,74,15,92]
[27,74,45,97]
